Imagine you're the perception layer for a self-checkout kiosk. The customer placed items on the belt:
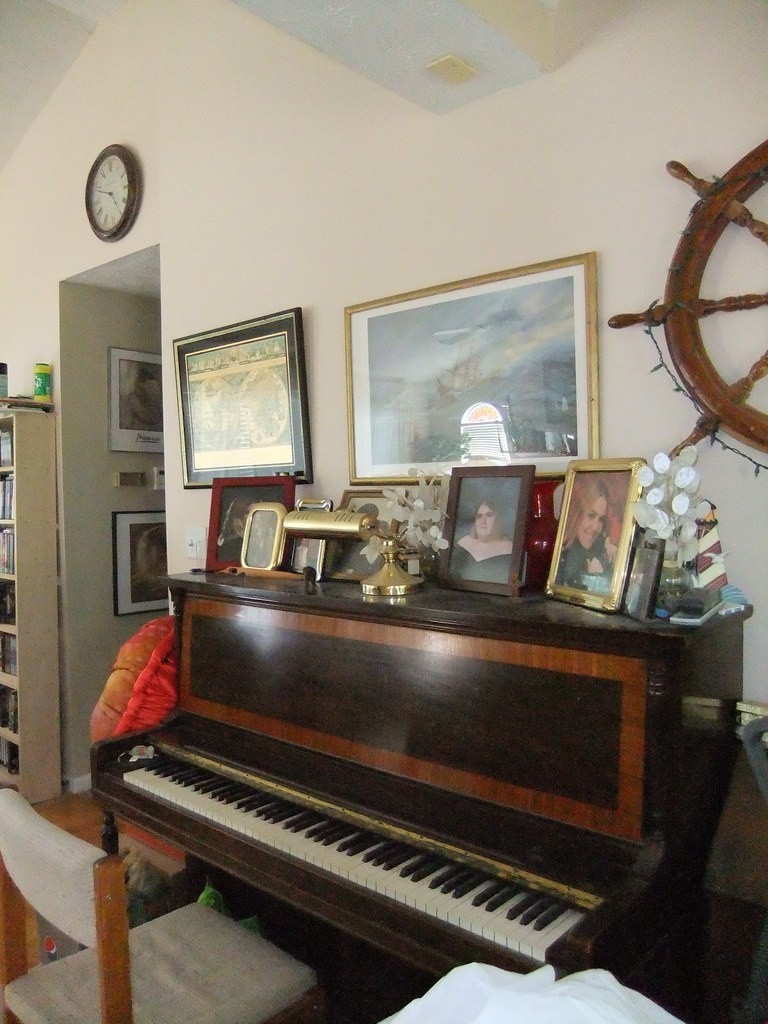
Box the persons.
[216,497,264,564]
[294,538,308,573]
[555,480,613,591]
[448,497,513,584]
[247,513,274,563]
[338,520,389,578]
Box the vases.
[394,546,440,584]
[654,560,693,619]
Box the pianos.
[91,563,758,1024]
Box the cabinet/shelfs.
[0,412,61,805]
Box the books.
[0,685,17,734]
[0,527,15,576]
[0,473,15,520]
[0,632,17,678]
[0,737,18,775]
[0,583,15,625]
[0,426,14,468]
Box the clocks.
[84,142,144,242]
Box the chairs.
[700,733,768,1024]
[0,788,322,1023]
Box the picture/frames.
[323,488,408,583]
[545,457,647,613]
[343,249,602,487]
[287,499,333,583]
[111,509,169,616]
[106,346,163,455]
[172,308,314,491]
[242,501,287,570]
[205,475,295,574]
[435,464,535,598]
